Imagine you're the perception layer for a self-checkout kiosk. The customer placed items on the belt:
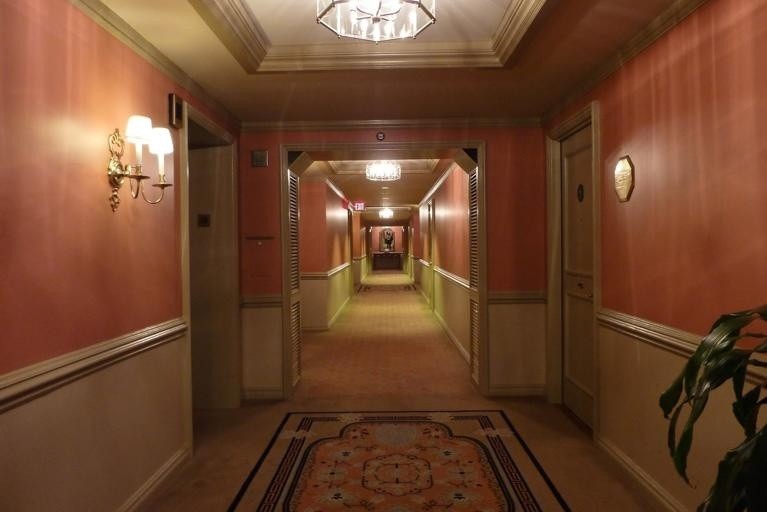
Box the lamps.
[365,160,401,219]
[316,0,438,47]
[109,115,174,213]
